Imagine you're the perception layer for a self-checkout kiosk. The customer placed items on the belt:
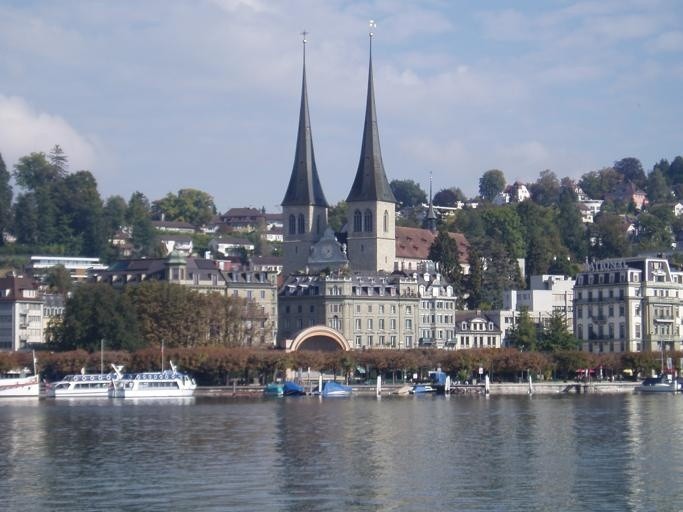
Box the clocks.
[319,244,335,259]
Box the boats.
[634,371,682,391]
[392,383,437,396]
[46,367,108,397]
[310,382,351,398]
[108,360,197,398]
[264,380,305,396]
[0,374,40,397]
